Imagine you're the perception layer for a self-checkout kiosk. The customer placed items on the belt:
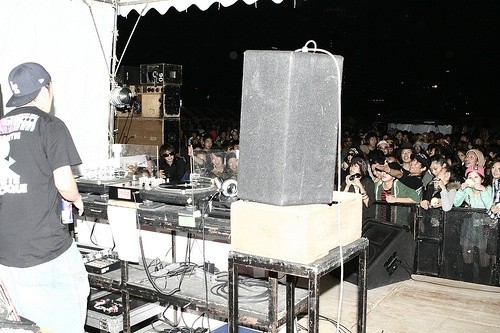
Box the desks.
[228,237,369,333]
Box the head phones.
[213,177,238,197]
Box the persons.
[154,143,191,181]
[0,63,90,333]
[335,127,500,285]
[188,126,239,192]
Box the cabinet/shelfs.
[68,191,309,333]
[115,117,181,158]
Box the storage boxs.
[85,292,159,333]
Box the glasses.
[347,153,354,155]
[163,151,175,157]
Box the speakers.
[115,63,183,161]
[236,49,344,205]
[330,216,417,289]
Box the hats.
[411,152,428,166]
[466,150,485,167]
[465,165,485,178]
[6,62,52,107]
[377,141,389,148]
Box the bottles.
[61,198,73,224]
